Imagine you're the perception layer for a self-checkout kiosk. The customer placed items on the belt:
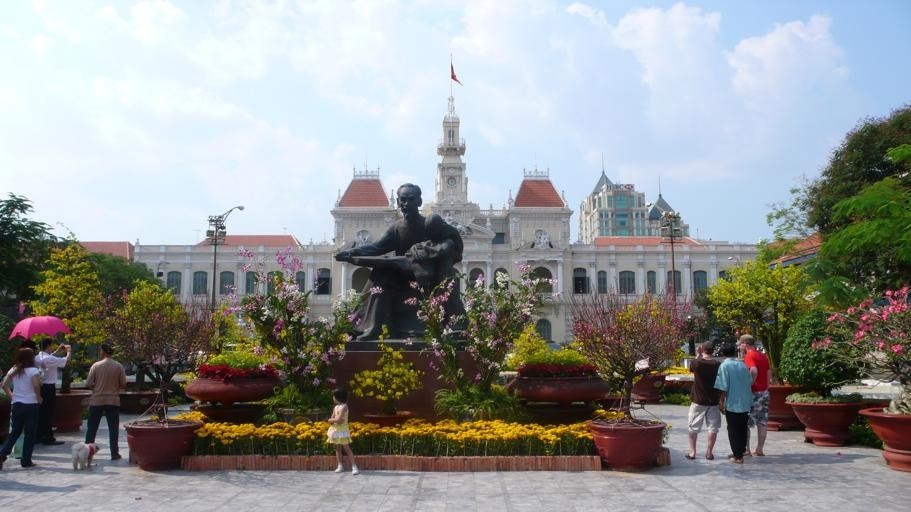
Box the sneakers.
[728,454,744,464]
[352,464,359,476]
[334,464,344,473]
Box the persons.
[0,347,44,472]
[326,389,359,475]
[34,337,71,445]
[9,340,36,459]
[85,343,127,460]
[714,345,754,465]
[335,184,468,341]
[739,334,771,455]
[684,341,721,461]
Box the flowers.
[560,286,706,423]
[813,286,911,414]
[91,287,221,423]
[349,329,425,412]
[519,350,599,377]
[196,354,281,379]
[404,259,561,405]
[225,245,384,405]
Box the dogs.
[71,442,100,472]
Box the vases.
[118,388,173,414]
[186,378,287,402]
[860,407,911,473]
[450,402,513,419]
[364,411,414,423]
[274,407,327,422]
[515,375,610,404]
[123,419,199,472]
[632,372,668,402]
[585,418,667,470]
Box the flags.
[451,63,463,87]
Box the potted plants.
[27,243,107,431]
[707,253,817,429]
[781,314,867,446]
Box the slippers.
[704,453,715,460]
[684,453,696,460]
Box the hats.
[735,334,755,345]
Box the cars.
[223,344,237,353]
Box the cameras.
[61,346,65,349]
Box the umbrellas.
[11,316,72,342]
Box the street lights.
[205,205,245,359]
[647,202,681,367]
[686,315,696,356]
[728,256,740,264]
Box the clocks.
[448,178,457,186]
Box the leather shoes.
[44,440,65,446]
